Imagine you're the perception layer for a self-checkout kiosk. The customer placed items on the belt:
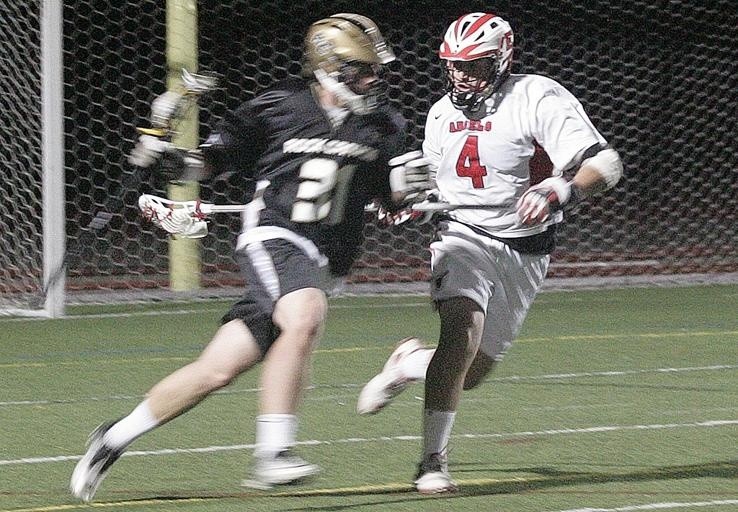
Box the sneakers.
[252,447,318,484]
[70,420,121,502]
[414,453,451,495]
[358,336,426,415]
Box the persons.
[359,12,623,494]
[70,11,433,500]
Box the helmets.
[300,13,396,79]
[439,12,514,92]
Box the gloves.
[388,150,437,205]
[515,177,572,227]
[127,135,183,180]
